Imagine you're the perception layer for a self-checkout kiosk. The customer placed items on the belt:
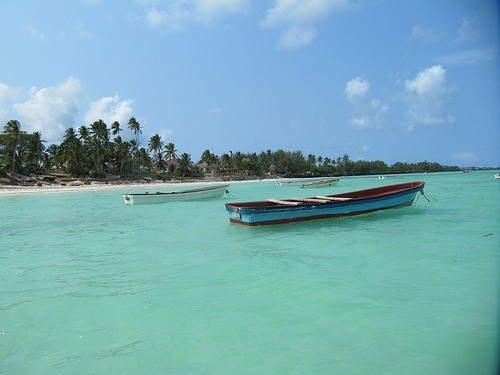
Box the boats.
[493,173,500,179]
[7,172,31,183]
[464,169,470,173]
[225,181,425,226]
[28,174,57,182]
[299,179,339,188]
[122,183,230,205]
